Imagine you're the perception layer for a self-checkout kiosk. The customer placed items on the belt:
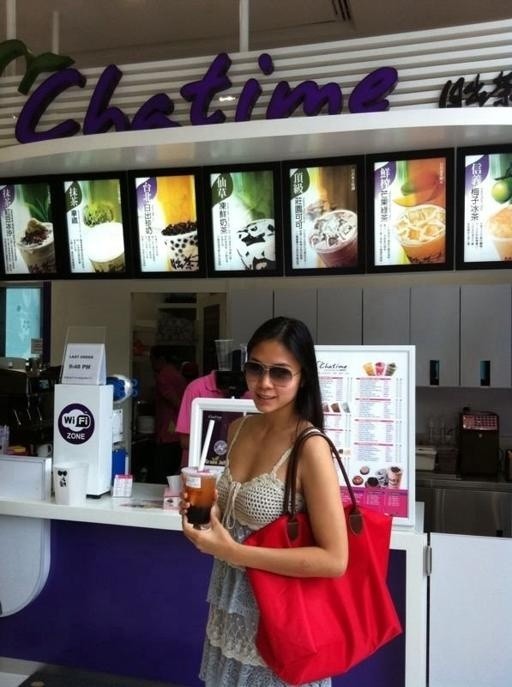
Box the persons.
[178,317,350,687]
[148,346,192,444]
[175,350,251,466]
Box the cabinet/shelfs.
[128,292,221,486]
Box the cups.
[396,205,445,263]
[489,212,512,262]
[181,467,220,525]
[163,230,198,270]
[214,338,235,371]
[37,444,52,459]
[240,343,249,372]
[234,218,276,270]
[82,222,124,274]
[17,223,54,273]
[362,361,396,376]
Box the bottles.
[388,468,402,488]
[307,210,358,268]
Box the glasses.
[243,361,302,384]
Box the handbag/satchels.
[243,425,402,685]
